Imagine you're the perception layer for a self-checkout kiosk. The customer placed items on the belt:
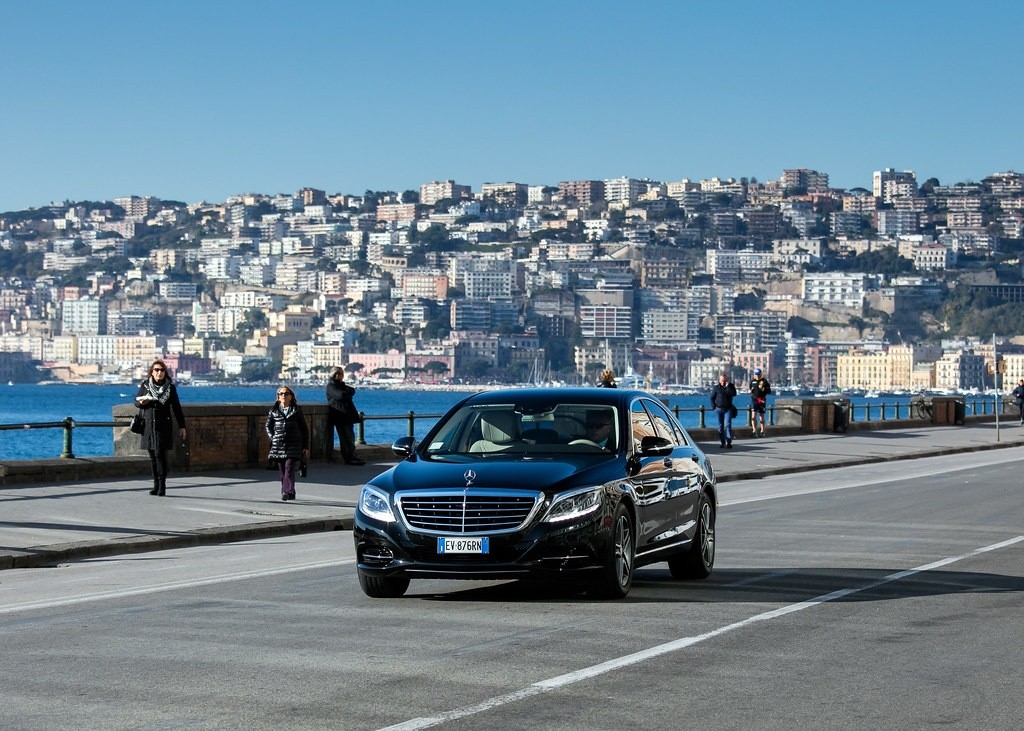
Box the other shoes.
[727,444,733,448]
[288,493,295,500]
[281,493,288,500]
[760,432,765,437]
[720,443,726,448]
[751,432,758,438]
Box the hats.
[754,368,761,375]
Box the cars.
[352,386,720,598]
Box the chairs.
[525,429,559,444]
[469,411,523,453]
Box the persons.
[1012,379,1024,425]
[561,408,616,457]
[711,374,737,449]
[325,366,367,466]
[264,386,310,501]
[598,371,617,388]
[749,367,771,436]
[135,360,186,497]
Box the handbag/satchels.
[298,451,307,477]
[756,396,766,405]
[130,408,145,434]
[731,406,737,418]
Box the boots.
[149,482,158,494]
[157,482,166,495]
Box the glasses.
[588,422,609,430]
[278,392,289,396]
[153,367,165,372]
